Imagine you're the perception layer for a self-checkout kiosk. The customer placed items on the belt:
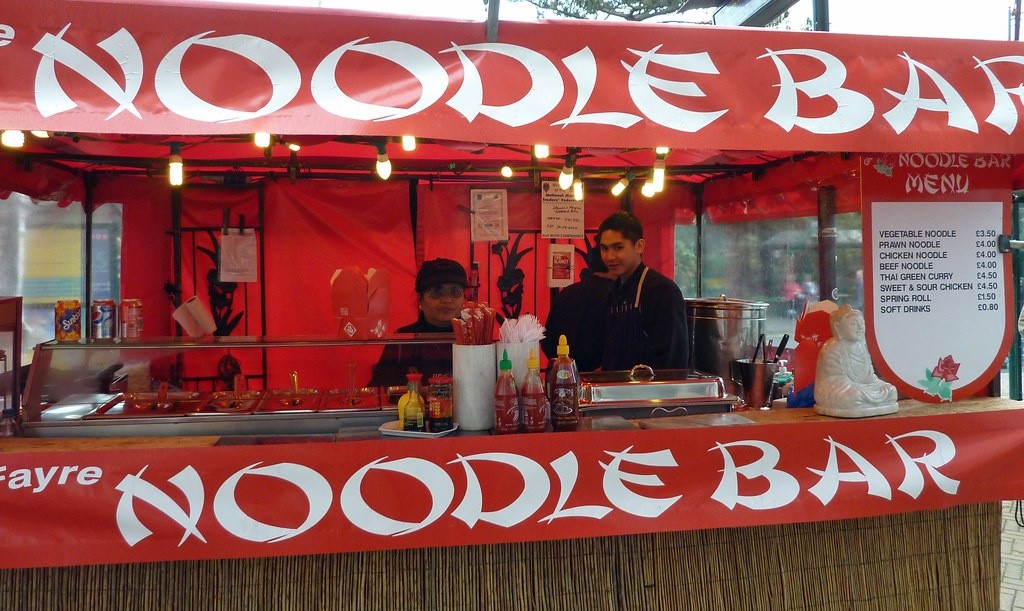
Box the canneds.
[117,299,144,344]
[90,299,117,343]
[54,299,81,342]
[427,376,453,433]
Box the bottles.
[427,376,455,433]
[0,409,20,439]
[495,349,520,435]
[551,335,582,432]
[518,348,550,434]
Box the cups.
[497,343,540,390]
[452,341,496,430]
[398,382,425,431]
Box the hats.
[415,258,467,292]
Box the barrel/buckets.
[683,294,769,402]
[736,359,778,406]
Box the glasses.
[427,289,463,298]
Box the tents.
[762,228,862,272]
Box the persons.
[540,210,689,371]
[854,269,864,310]
[366,258,469,387]
[784,272,819,320]
[814,303,897,410]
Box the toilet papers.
[175,299,218,338]
